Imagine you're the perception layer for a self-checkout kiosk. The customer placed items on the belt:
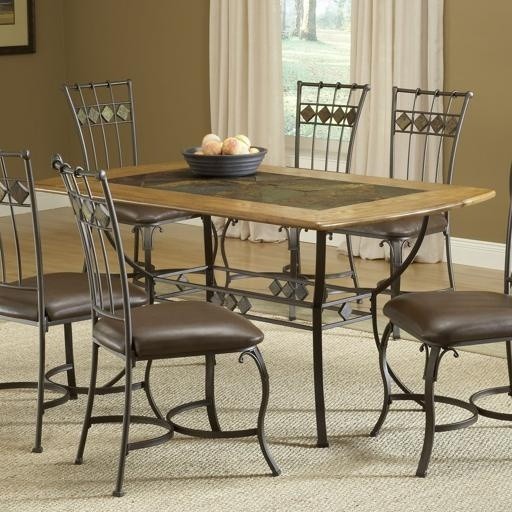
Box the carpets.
[1,298,512,512]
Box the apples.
[194,134,259,156]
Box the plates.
[182,147,267,178]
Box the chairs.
[370,164,512,476]
[51,152,282,495]
[289,86,474,339]
[63,78,215,304]
[221,81,372,323]
[1,149,165,453]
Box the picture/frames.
[0,0,37,55]
